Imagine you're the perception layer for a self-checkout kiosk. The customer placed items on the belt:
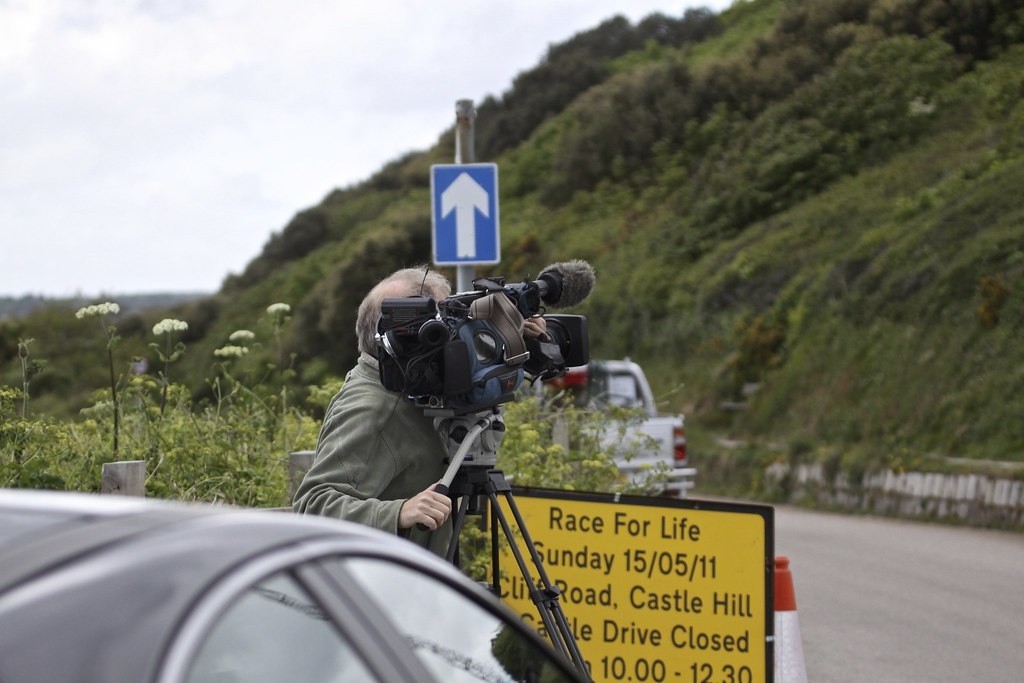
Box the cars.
[0,487,598,683]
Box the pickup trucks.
[568,353,696,503]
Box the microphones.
[448,259,595,313]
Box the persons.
[291,267,553,560]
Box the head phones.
[375,313,450,360]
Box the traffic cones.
[769,555,808,683]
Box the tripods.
[416,404,594,683]
